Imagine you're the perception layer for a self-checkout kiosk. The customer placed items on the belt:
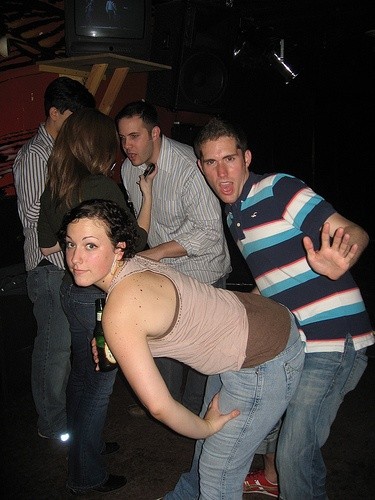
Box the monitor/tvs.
[63,0,153,61]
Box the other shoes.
[71,475,128,494]
[128,406,146,417]
[102,435,121,455]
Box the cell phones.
[143,162,154,176]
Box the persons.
[38,107,157,496]
[194,110,375,500]
[12,78,97,439]
[65,200,305,500]
[116,105,230,437]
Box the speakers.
[144,1,242,115]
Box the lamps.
[268,37,300,87]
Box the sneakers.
[242,469,279,498]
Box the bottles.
[93,298,118,373]
